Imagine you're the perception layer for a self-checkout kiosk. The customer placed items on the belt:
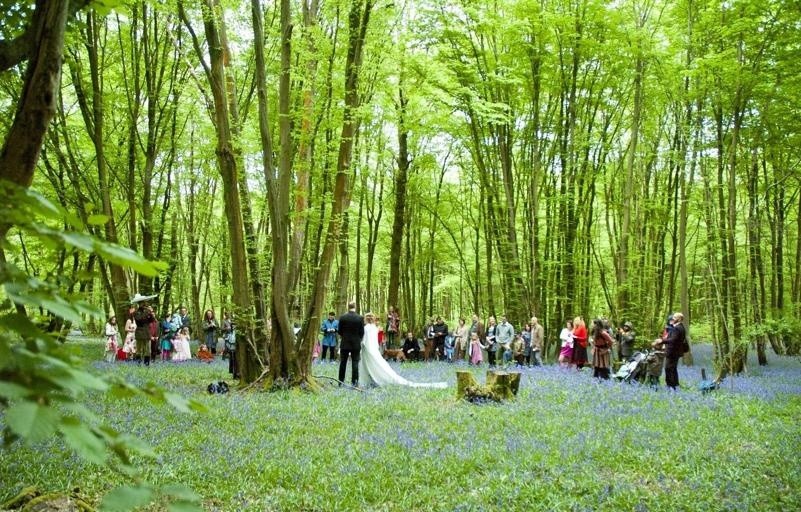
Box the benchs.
[382,348,466,362]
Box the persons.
[195,310,235,361]
[339,302,365,388]
[375,306,456,362]
[105,316,120,362]
[359,313,448,389]
[455,314,542,366]
[657,312,691,391]
[122,302,191,365]
[559,316,635,384]
[313,311,340,363]
